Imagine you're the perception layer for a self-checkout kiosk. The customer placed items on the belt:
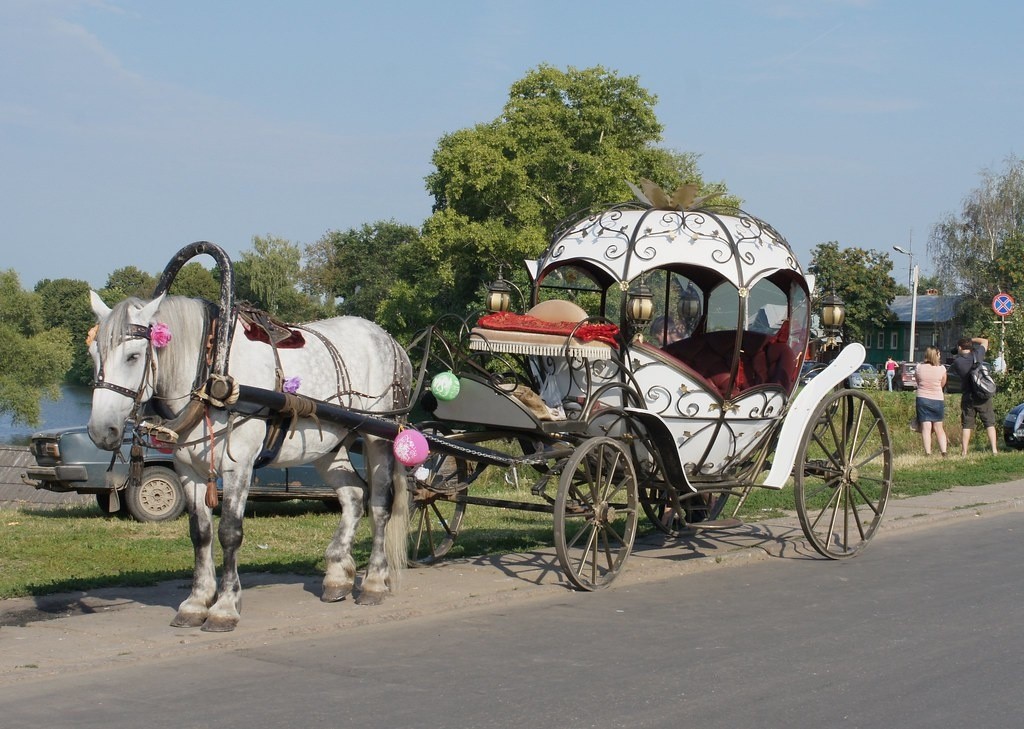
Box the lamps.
[485,263,527,317]
[676,279,701,327]
[626,270,655,335]
[821,283,845,338]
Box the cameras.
[950,344,960,355]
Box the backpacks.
[969,349,996,400]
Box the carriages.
[85,176,895,633]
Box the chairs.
[658,327,801,406]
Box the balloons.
[393,429,429,466]
[431,370,460,400]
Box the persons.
[884,354,900,391]
[649,315,719,532]
[948,336,999,455]
[914,344,949,456]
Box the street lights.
[891,244,918,363]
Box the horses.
[87,289,413,634]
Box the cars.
[883,361,920,388]
[25,397,441,523]
[797,358,820,386]
[844,363,881,390]
[939,364,965,393]
